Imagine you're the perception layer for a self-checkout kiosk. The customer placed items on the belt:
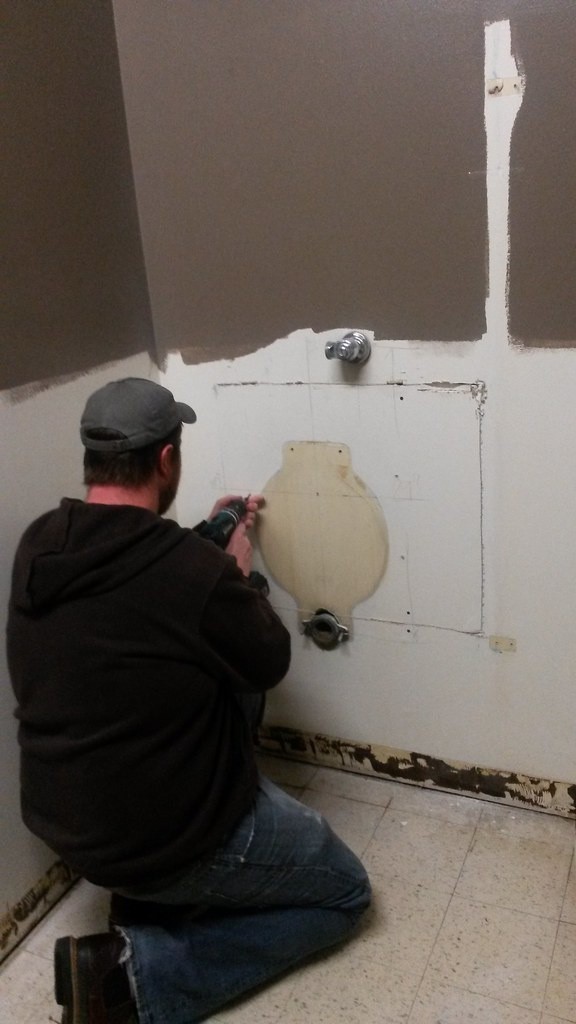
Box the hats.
[80,376,196,451]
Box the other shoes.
[54,932,136,1024]
[108,893,174,930]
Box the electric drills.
[191,494,251,549]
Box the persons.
[8,376,372,1024]
